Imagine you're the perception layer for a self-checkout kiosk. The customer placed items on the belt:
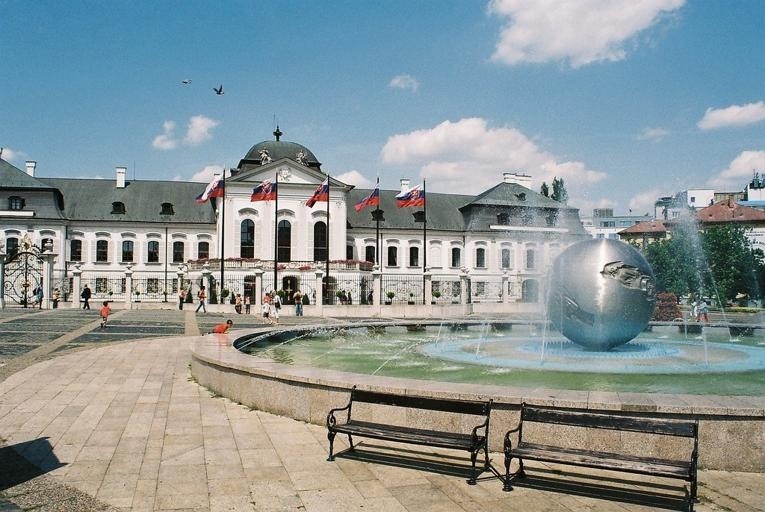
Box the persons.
[233,293,242,314]
[178,286,185,310]
[347,292,353,305]
[688,298,698,321]
[99,301,112,328]
[367,290,373,305]
[201,319,233,337]
[36,286,43,310]
[245,293,250,314]
[696,299,710,321]
[312,289,316,306]
[52,287,60,309]
[292,289,303,316]
[339,289,347,305]
[80,283,91,310]
[30,287,37,308]
[194,286,209,315]
[262,289,283,324]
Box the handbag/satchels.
[275,301,280,308]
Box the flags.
[250,174,276,202]
[193,171,224,205]
[354,182,380,215]
[305,176,329,209]
[394,182,425,210]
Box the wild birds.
[213,84,224,96]
[182,79,191,84]
